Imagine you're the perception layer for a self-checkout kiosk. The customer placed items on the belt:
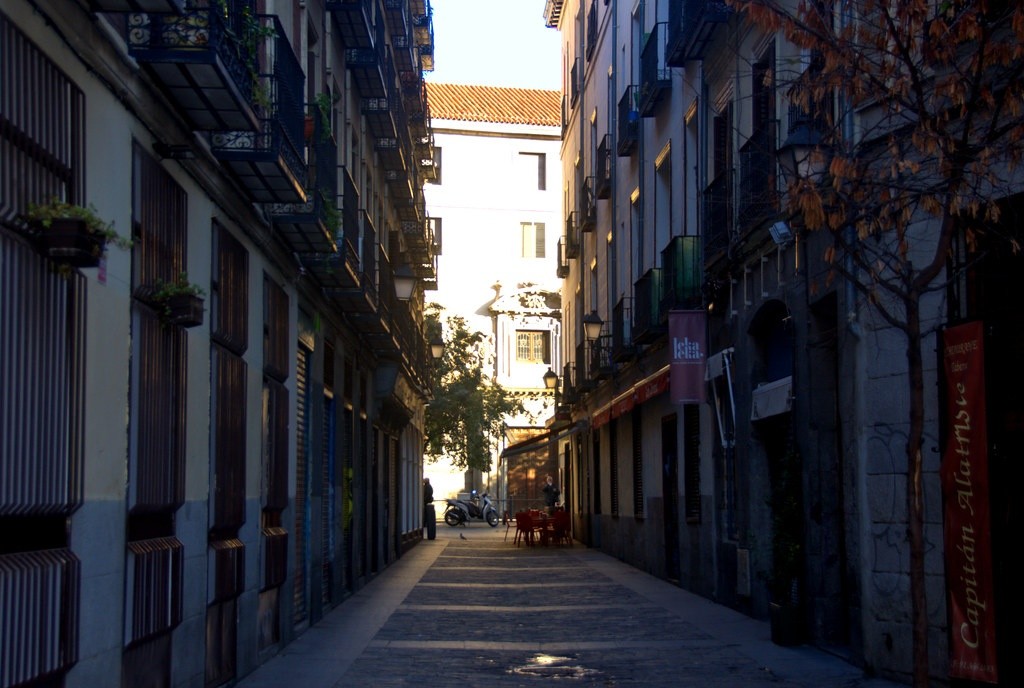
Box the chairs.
[559,529,573,546]
[504,511,517,542]
[514,512,534,547]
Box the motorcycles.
[442,491,500,528]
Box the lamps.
[582,309,603,342]
[428,333,446,361]
[543,367,559,388]
[391,262,420,304]
[773,112,836,189]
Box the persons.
[544,476,561,514]
[423,478,434,506]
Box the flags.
[667,308,709,406]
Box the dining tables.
[530,518,560,546]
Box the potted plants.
[138,269,206,328]
[21,192,136,278]
